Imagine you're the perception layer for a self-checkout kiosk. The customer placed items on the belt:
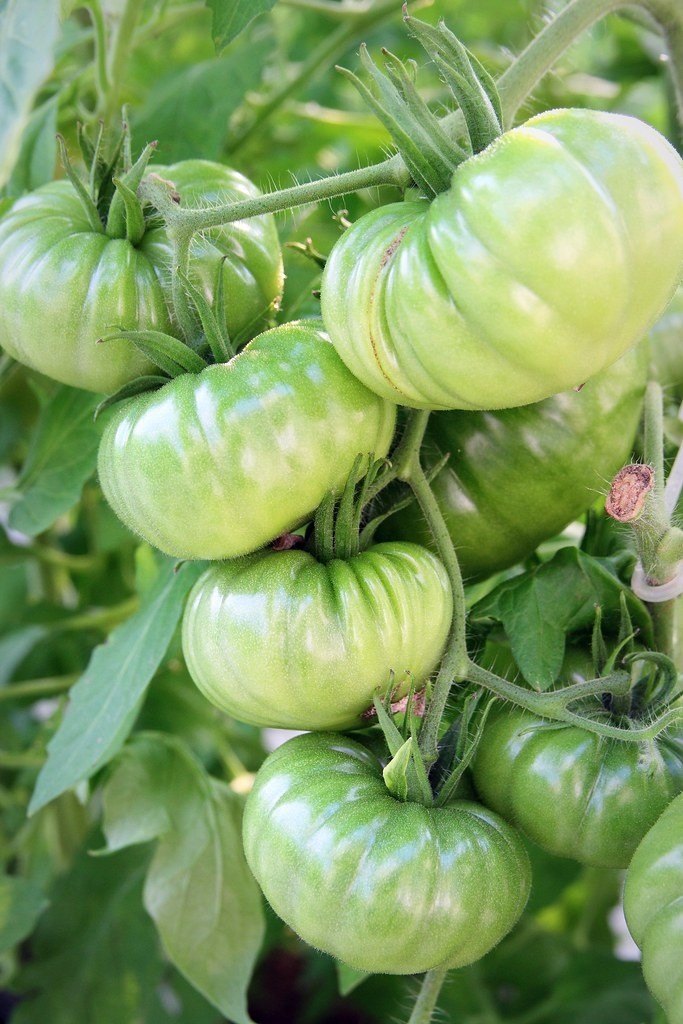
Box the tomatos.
[0,104,683,1024]
